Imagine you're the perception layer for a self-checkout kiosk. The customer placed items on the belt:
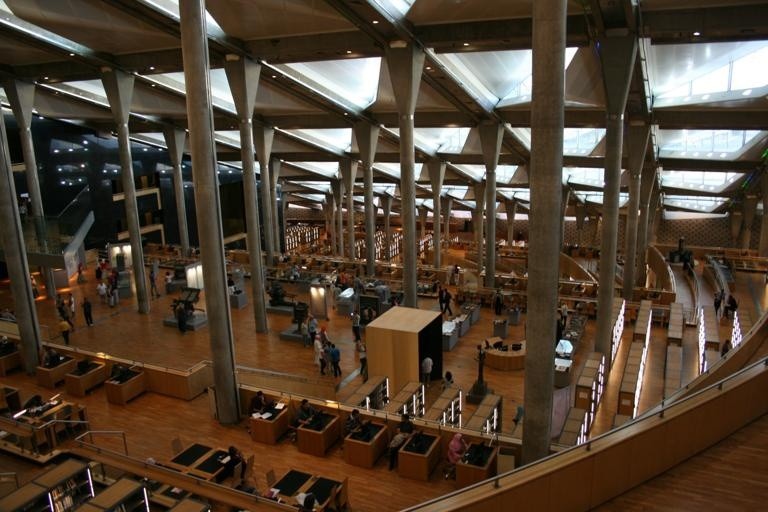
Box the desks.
[555,279,678,325]
[250,373,503,486]
[1,342,148,439]
[1,445,350,512]
[478,336,526,371]
[264,256,531,312]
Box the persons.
[300,269,368,383]
[149,271,161,297]
[438,286,443,311]
[42,347,57,362]
[422,355,435,384]
[391,297,400,306]
[560,303,568,327]
[557,319,565,344]
[714,294,720,314]
[250,391,468,482]
[24,395,43,409]
[442,371,454,391]
[164,271,172,294]
[495,289,502,315]
[453,265,459,286]
[176,304,187,333]
[721,340,729,357]
[227,275,234,287]
[724,295,738,317]
[443,288,452,316]
[224,446,247,478]
[56,263,119,345]
